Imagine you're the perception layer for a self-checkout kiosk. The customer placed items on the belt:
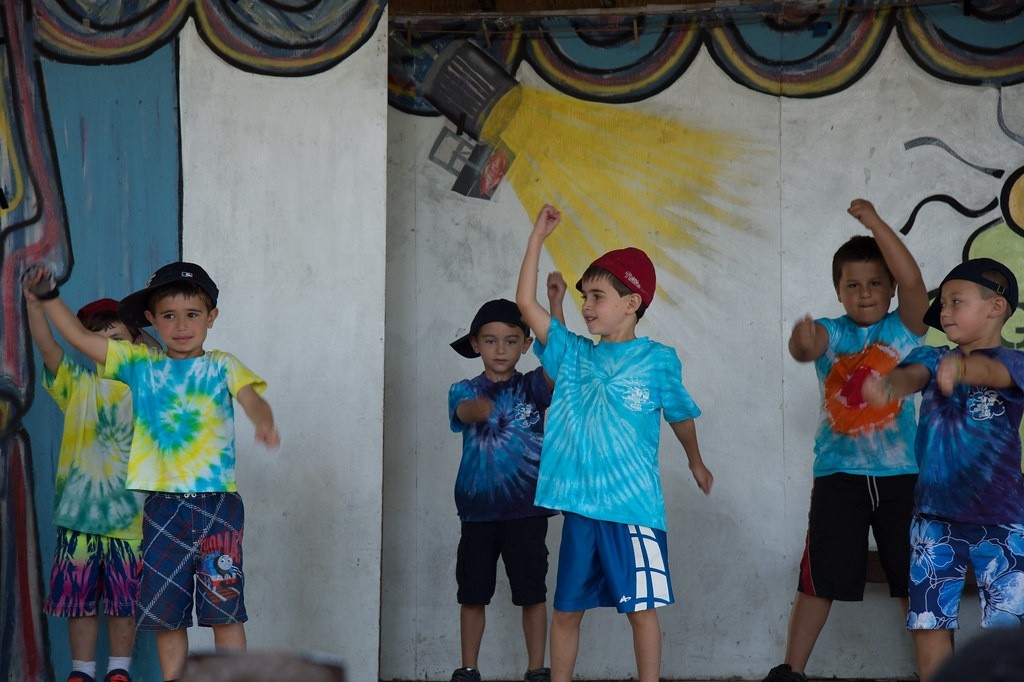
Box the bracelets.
[953,354,966,384]
[880,375,893,402]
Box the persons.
[447,271,567,682]
[517,204,715,682]
[760,196,1024,682]
[18,262,281,682]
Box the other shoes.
[524,667,552,682]
[449,667,481,682]
[761,664,806,682]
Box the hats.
[450,299,530,359]
[75,297,163,351]
[575,248,656,308]
[118,261,219,329]
[922,258,1019,334]
